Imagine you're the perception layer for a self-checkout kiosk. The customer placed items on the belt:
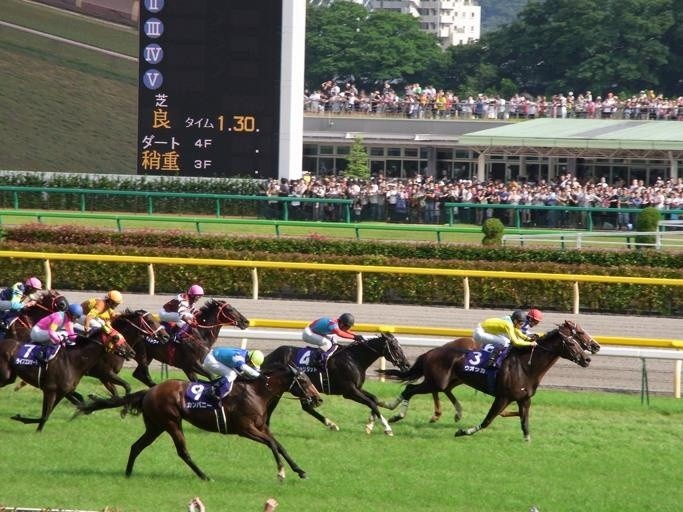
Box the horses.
[430,319,600,423]
[0,319,136,431]
[260,331,411,436]
[14,308,170,397]
[66,360,323,483]
[132,299,249,389]
[373,323,593,443]
[1,289,69,343]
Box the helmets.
[68,304,83,319]
[247,348,264,367]
[189,284,205,295]
[512,310,528,322]
[528,309,542,321]
[340,313,354,326]
[26,277,43,290]
[107,290,123,304]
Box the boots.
[486,352,497,367]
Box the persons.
[258,174,485,225]
[508,89,683,122]
[475,309,538,372]
[30,303,82,366]
[303,81,506,120]
[160,285,204,343]
[76,290,123,334]
[481,174,683,231]
[204,349,264,411]
[0,278,43,327]
[506,311,542,351]
[301,314,360,369]
